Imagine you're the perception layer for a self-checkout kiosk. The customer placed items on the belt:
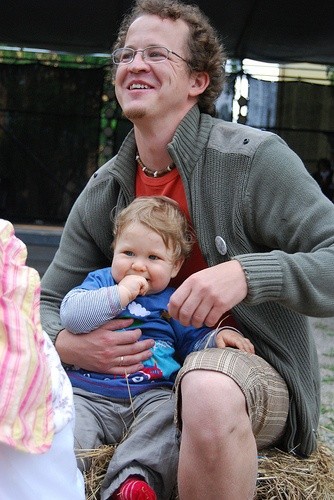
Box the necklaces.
[136,153,176,178]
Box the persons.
[60,194,256,500]
[312,159,334,202]
[40,0,334,500]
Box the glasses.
[111,47,196,67]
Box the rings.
[119,356,125,367]
